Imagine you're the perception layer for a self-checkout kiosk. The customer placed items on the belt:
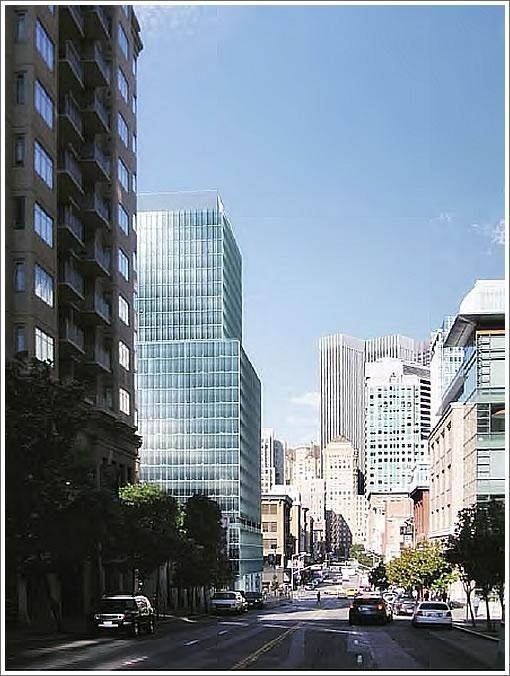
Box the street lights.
[290,552,312,603]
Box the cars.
[89,592,160,638]
[325,584,371,599]
[304,573,341,590]
[348,597,389,625]
[384,593,453,629]
[206,590,262,616]
